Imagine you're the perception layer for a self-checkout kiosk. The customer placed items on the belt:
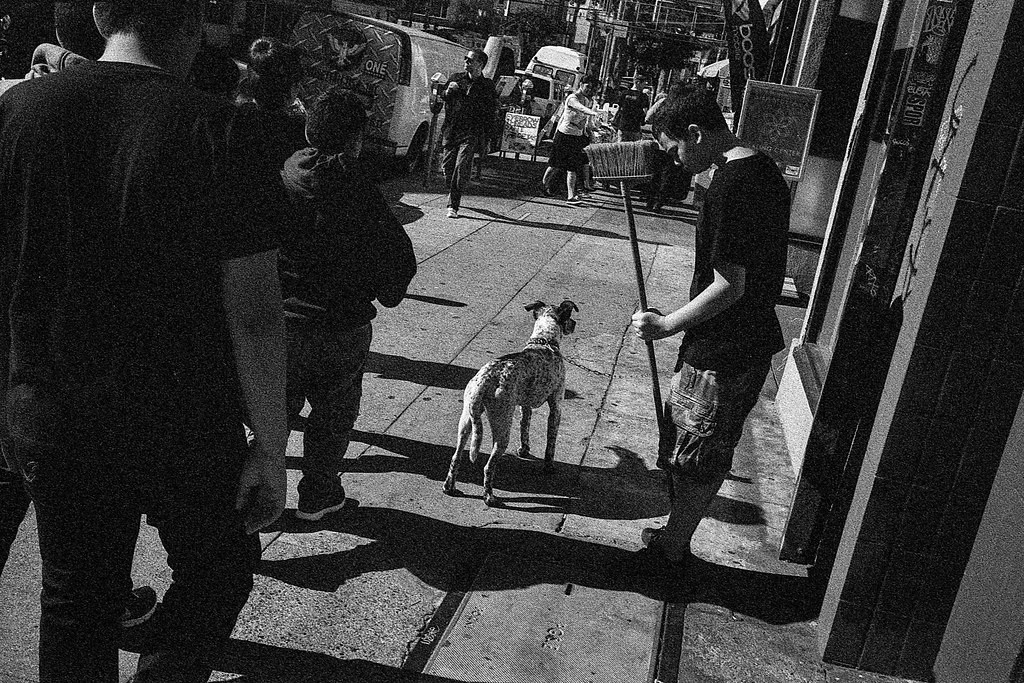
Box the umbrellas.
[697,59,731,112]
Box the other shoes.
[537,183,554,197]
[641,526,667,546]
[446,207,459,218]
[566,196,583,205]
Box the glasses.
[464,56,475,64]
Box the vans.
[509,46,591,131]
[268,0,483,178]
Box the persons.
[626,83,794,596]
[514,71,698,211]
[254,87,417,522]
[439,48,498,219]
[0,0,352,682]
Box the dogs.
[442,299,581,507]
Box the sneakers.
[120,586,158,627]
[295,487,346,521]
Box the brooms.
[582,140,664,425]
